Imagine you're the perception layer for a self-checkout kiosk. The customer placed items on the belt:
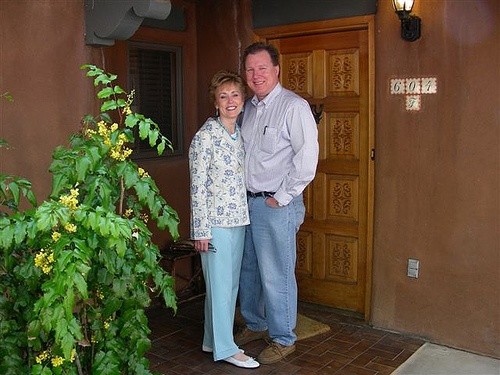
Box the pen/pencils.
[263,125,267,135]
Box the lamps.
[391,0,422,43]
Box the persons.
[189,70,260,369]
[234,42,320,364]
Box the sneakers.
[258,342,296,363]
[234,326,268,345]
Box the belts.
[247,191,275,197]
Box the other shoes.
[223,352,261,369]
[201,344,245,353]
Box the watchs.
[276,201,284,207]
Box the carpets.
[235,297,331,345]
